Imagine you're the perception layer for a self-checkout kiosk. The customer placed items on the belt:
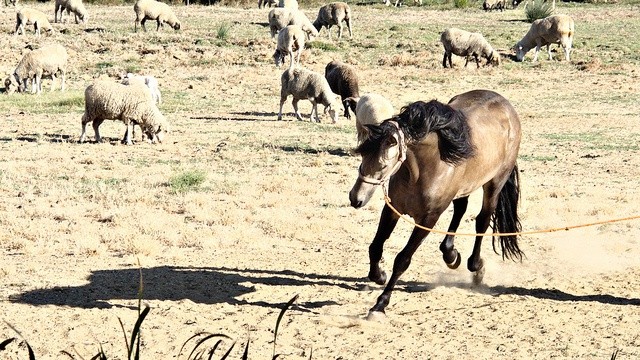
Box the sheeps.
[258,0,279,9]
[510,14,574,63]
[290,9,318,44]
[483,0,508,12]
[134,0,182,33]
[4,43,67,96]
[14,7,56,39]
[325,61,361,120]
[55,0,89,24]
[268,8,297,38]
[313,2,352,43]
[438,27,501,68]
[278,67,346,123]
[4,0,19,7]
[343,93,395,147]
[121,73,162,141]
[272,25,305,68]
[382,0,422,8]
[79,74,170,145]
[279,1,299,9]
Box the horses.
[349,90,528,321]
